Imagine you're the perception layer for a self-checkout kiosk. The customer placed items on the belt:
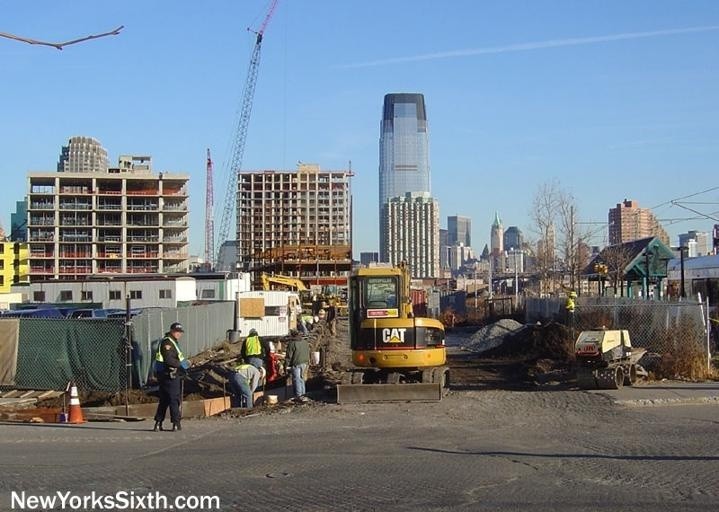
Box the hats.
[171,323,183,332]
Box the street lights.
[676,245,689,298]
[642,250,655,299]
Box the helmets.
[259,366,267,381]
[314,316,319,323]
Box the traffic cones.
[66,381,89,425]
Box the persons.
[240,329,270,369]
[229,365,266,409]
[284,331,310,400]
[153,322,192,433]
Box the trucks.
[2,308,141,319]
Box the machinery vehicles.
[259,269,348,326]
[571,325,638,389]
[335,258,451,405]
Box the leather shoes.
[155,421,162,432]
[173,424,182,432]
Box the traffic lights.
[593,262,599,274]
[600,265,610,275]
